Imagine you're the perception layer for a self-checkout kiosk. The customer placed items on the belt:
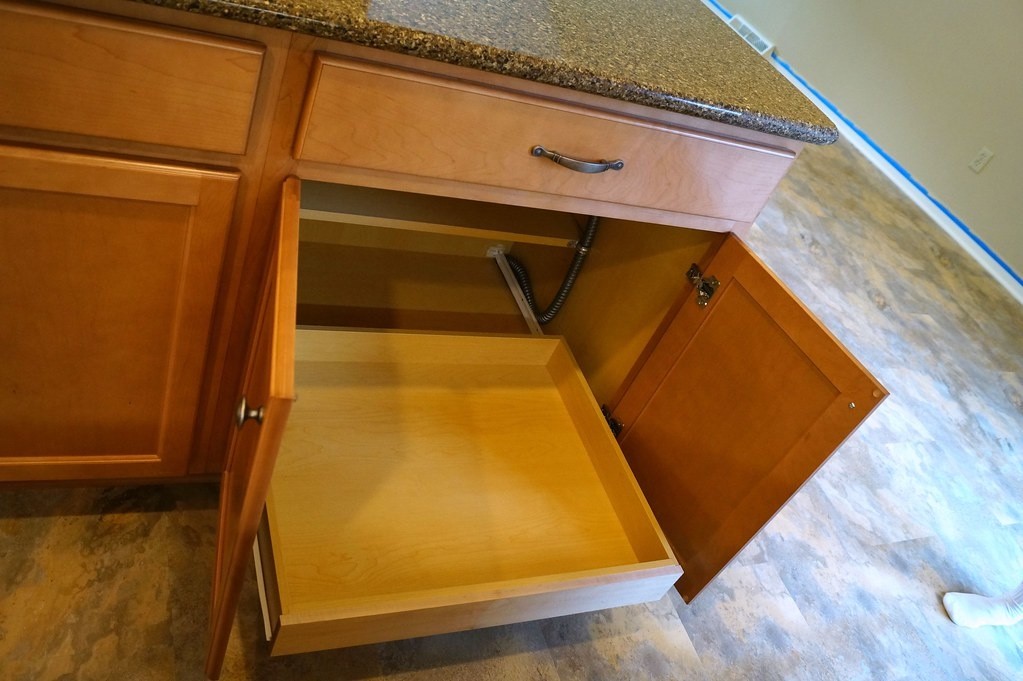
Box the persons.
[943,581,1023,630]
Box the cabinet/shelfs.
[205,10,891,680]
[0,0,293,494]
